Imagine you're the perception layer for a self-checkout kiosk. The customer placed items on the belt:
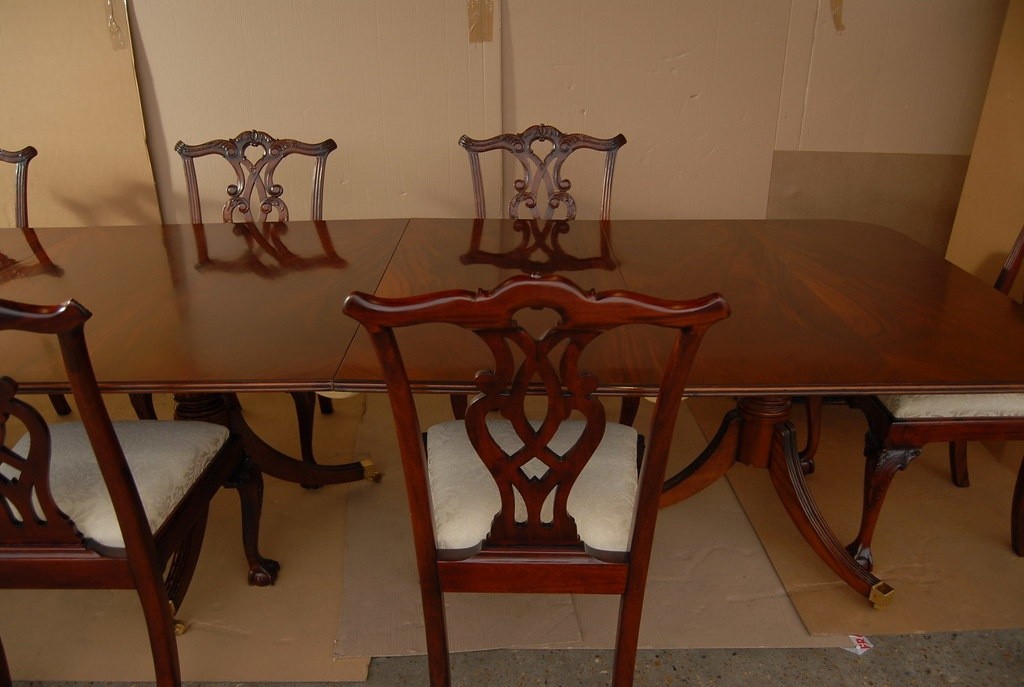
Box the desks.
[1,219,1023,605]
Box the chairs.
[343,273,730,687]
[947,225,1023,486]
[0,299,233,686]
[174,128,346,489]
[448,122,643,428]
[1,146,72,415]
